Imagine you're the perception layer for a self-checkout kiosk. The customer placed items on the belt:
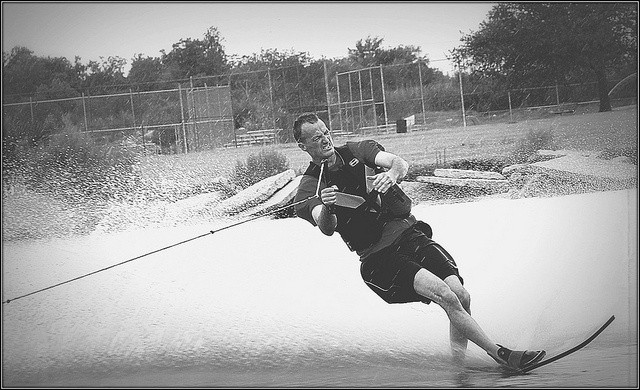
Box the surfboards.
[468,316,615,375]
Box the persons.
[293,112,545,368]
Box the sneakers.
[487,344,546,373]
